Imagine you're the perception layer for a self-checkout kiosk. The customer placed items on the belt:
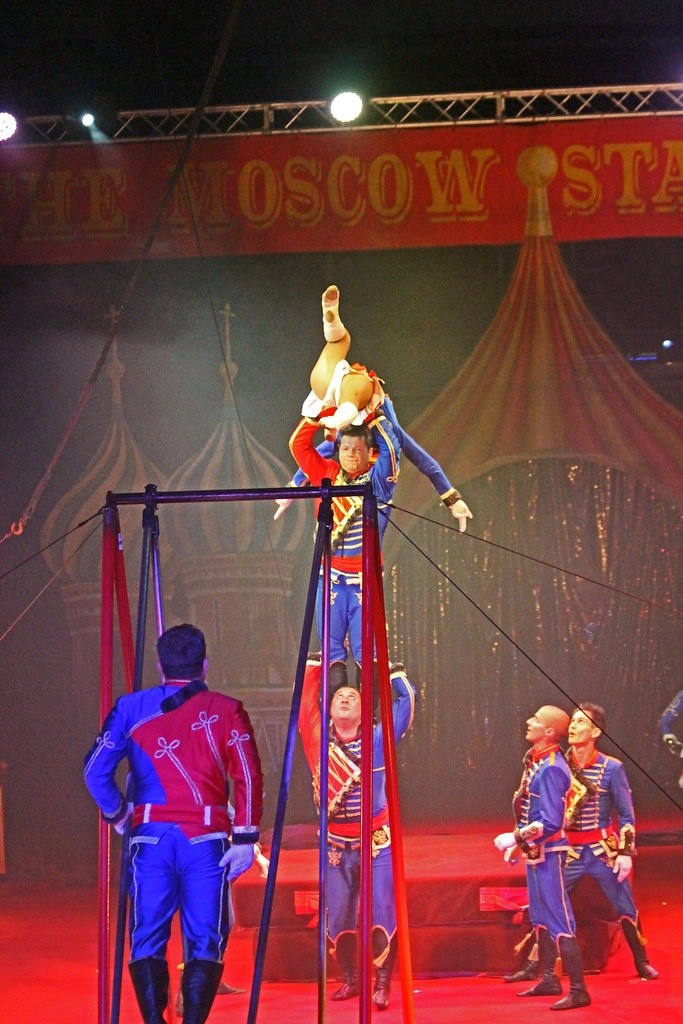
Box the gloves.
[662,734,683,758]
[612,854,632,884]
[504,843,518,862]
[494,831,516,852]
[218,826,260,884]
[449,500,473,533]
[273,480,295,520]
[100,795,134,836]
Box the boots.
[324,929,361,1001]
[516,925,562,997]
[329,660,348,695]
[358,657,380,728]
[550,933,590,1010]
[127,958,170,1024]
[180,958,225,1024]
[370,923,398,1008]
[503,927,540,983]
[617,910,658,980]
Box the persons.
[658,689,683,787]
[494,703,659,1011]
[297,650,416,1011]
[273,285,474,729]
[82,623,263,1024]
